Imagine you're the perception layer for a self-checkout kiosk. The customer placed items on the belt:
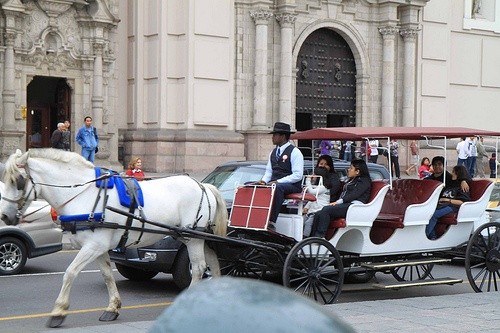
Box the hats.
[267,122,296,135]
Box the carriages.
[0,122,500,328]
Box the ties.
[276,148,280,160]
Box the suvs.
[108,157,390,291]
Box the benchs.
[439,177,496,224]
[327,180,390,228]
[375,178,446,228]
[283,175,324,213]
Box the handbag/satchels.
[94,127,98,153]
[378,144,384,155]
[384,150,388,156]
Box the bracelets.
[449,198,452,204]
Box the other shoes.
[267,224,276,231]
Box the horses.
[0,148,228,328]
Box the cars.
[0,198,63,276]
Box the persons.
[125,156,144,182]
[406,136,500,240]
[75,116,99,165]
[303,138,401,241]
[49,120,71,152]
[262,122,304,232]
